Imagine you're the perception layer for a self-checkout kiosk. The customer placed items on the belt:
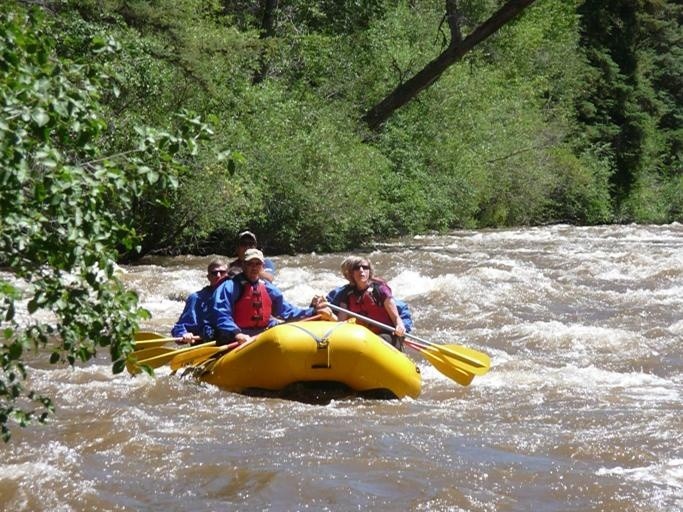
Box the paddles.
[171,313,322,371]
[402,339,475,387]
[130,331,202,351]
[325,303,490,375]
[128,341,216,375]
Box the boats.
[184,317,427,407]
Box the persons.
[171,231,412,358]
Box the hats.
[237,230,258,245]
[244,247,264,263]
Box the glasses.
[211,270,227,277]
[354,264,370,271]
